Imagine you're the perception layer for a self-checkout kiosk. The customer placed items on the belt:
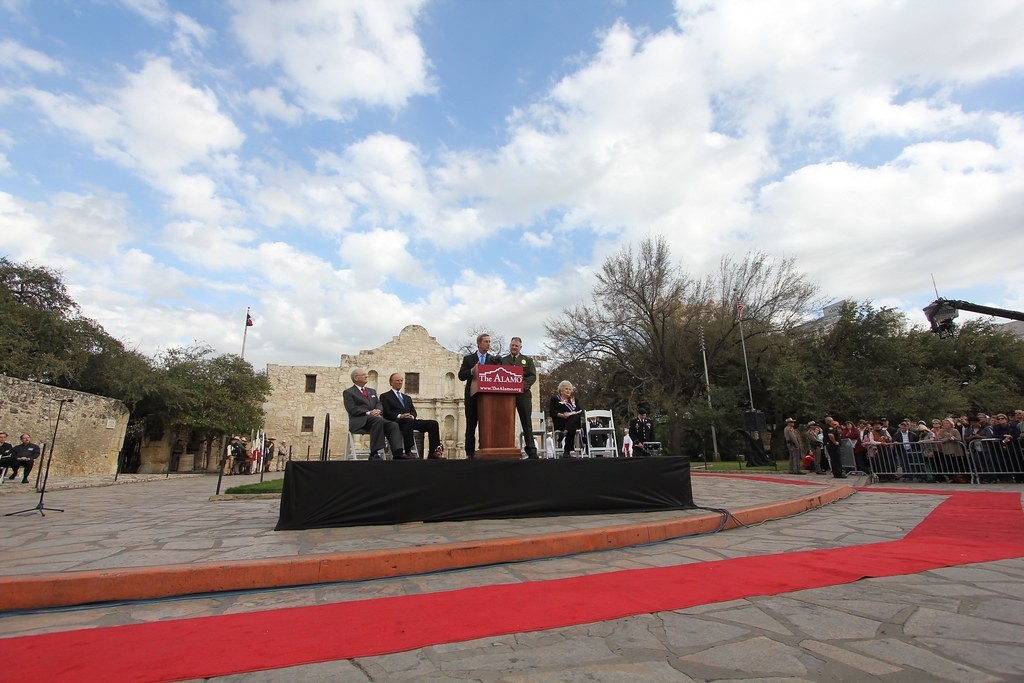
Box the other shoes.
[465,456,474,460]
[528,454,538,460]
[369,450,447,461]
[563,451,571,459]
[881,475,1022,484]
[9,472,17,479]
[22,477,29,483]
[557,432,564,443]
[795,468,855,478]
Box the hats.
[784,418,797,425]
[234,436,286,443]
[807,421,817,427]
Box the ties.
[480,354,485,364]
[512,356,516,362]
[361,387,368,397]
[396,391,405,408]
[641,418,644,424]
[593,422,595,426]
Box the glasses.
[356,373,367,377]
[1015,412,1022,414]
[997,417,1006,420]
[919,430,925,432]
[932,422,939,424]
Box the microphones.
[56,398,73,402]
[488,354,502,365]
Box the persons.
[803,409,1024,485]
[550,380,581,458]
[621,427,633,457]
[0,432,40,483]
[784,418,805,475]
[629,408,655,456]
[582,408,608,458]
[224,435,288,476]
[379,373,446,459]
[501,337,538,460]
[546,432,554,459]
[343,368,412,461]
[458,334,497,460]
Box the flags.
[736,299,743,320]
[246,315,255,326]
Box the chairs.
[518,411,547,459]
[584,409,618,458]
[551,421,584,460]
[344,412,420,460]
[0,466,25,484]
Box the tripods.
[4,401,64,517]
